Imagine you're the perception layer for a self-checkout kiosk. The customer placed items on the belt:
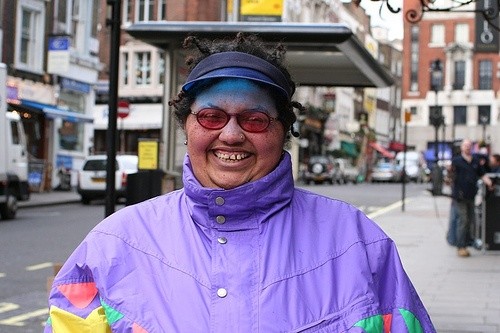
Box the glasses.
[189,110,279,133]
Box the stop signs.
[118,102,130,119]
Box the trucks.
[0,64,28,218]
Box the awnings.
[7,97,94,122]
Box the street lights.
[431,58,442,166]
[480,111,488,142]
[317,108,330,155]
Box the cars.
[371,163,402,181]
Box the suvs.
[303,156,359,185]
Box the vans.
[77,154,138,206]
[395,151,427,181]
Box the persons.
[446,137,500,256]
[42,31,436,333]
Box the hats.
[182,52,292,99]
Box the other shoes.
[456,248,470,258]
